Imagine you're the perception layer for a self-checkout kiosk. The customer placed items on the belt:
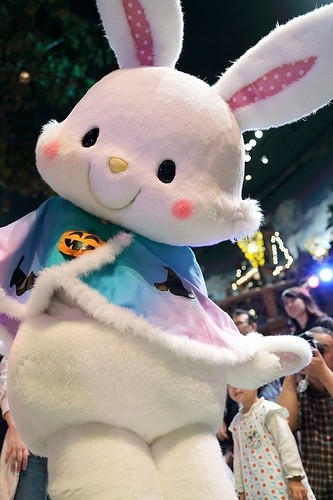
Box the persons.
[0,353,49,500]
[216,287,333,500]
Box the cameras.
[298,331,324,357]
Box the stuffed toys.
[0,0,333,500]
